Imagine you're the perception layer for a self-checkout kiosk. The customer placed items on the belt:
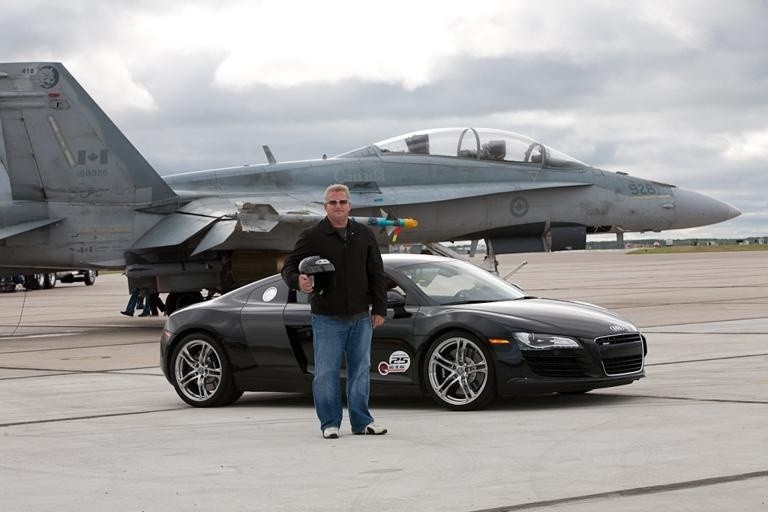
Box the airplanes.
[0,62,743,316]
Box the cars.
[160,253,647,410]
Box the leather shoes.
[121,306,167,317]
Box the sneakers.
[352,422,387,434]
[323,426,339,438]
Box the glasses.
[327,200,348,205]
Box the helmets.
[298,255,336,296]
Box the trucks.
[0,269,99,292]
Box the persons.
[120,289,165,317]
[280,183,389,439]
